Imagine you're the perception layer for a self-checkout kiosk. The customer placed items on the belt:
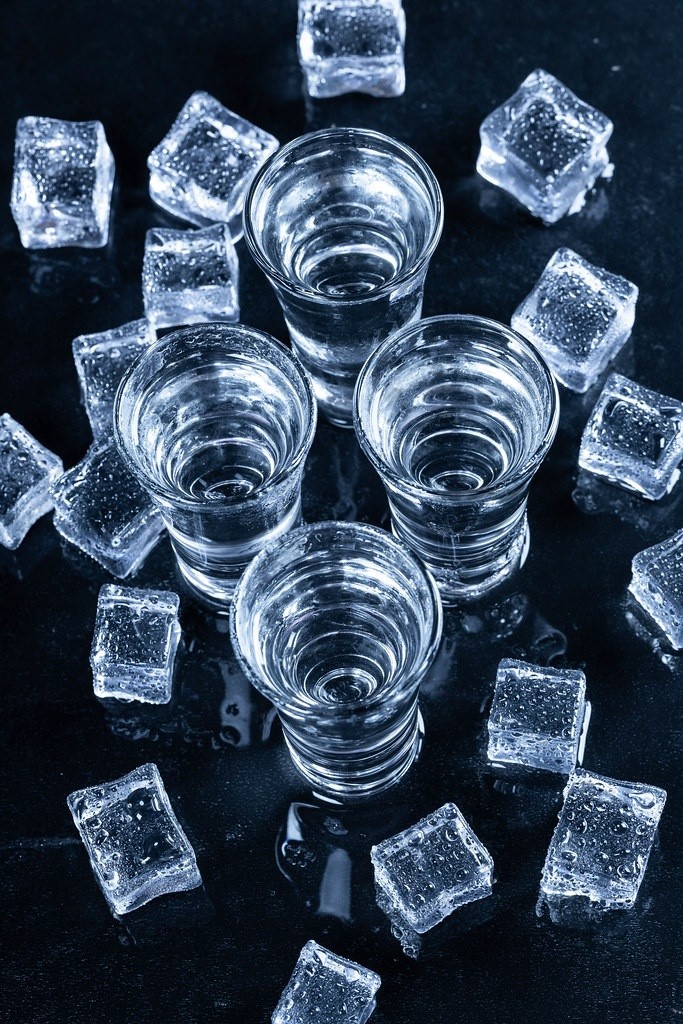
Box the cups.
[243,128,445,380]
[230,520,444,799]
[352,315,561,596]
[112,323,317,607]
